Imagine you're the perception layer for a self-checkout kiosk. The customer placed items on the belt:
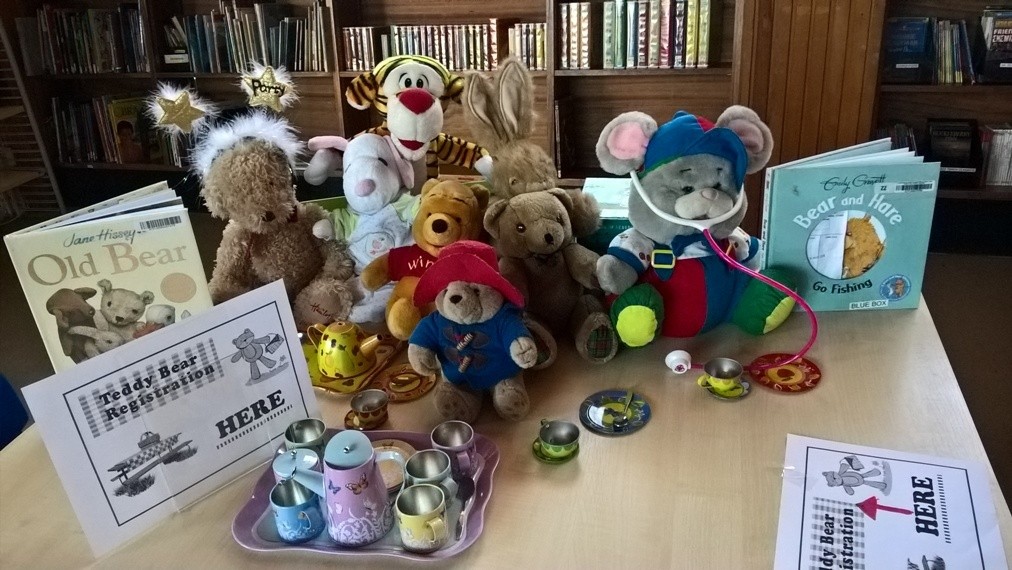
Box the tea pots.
[307,321,383,378]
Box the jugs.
[291,430,407,547]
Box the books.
[756,135,941,315]
[878,3,1012,193]
[340,10,547,70]
[554,0,722,75]
[2,179,215,379]
[9,0,333,175]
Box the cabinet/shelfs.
[0,0,1012,260]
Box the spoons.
[613,391,633,428]
[392,375,424,384]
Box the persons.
[117,119,144,164]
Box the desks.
[0,236,1012,570]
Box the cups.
[431,420,478,479]
[405,449,458,509]
[539,418,579,456]
[284,418,327,473]
[272,448,322,483]
[395,484,448,554]
[269,479,326,543]
[350,389,388,424]
[697,358,743,390]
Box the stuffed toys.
[482,186,619,370]
[406,240,539,425]
[302,124,433,323]
[360,177,495,339]
[594,103,807,350]
[460,62,604,251]
[341,50,496,199]
[143,55,356,336]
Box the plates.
[749,352,822,393]
[302,336,398,395]
[372,439,417,494]
[579,389,651,435]
[532,437,579,464]
[344,410,388,430]
[371,363,437,402]
[705,379,750,401]
